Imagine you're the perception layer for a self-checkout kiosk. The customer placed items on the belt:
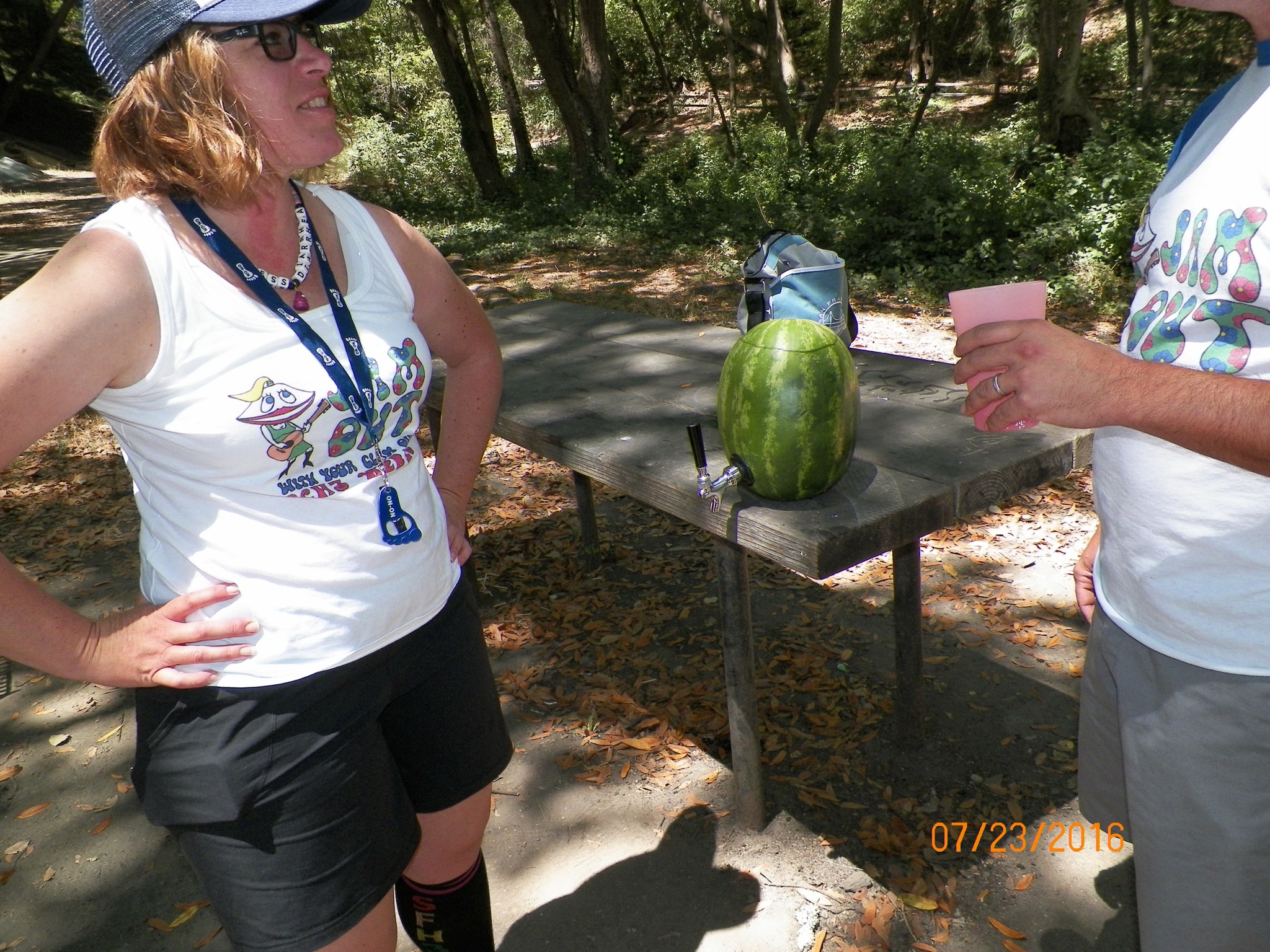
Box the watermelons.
[717,318,860,502]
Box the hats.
[82,0,373,98]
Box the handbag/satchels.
[737,230,858,350]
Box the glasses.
[206,22,323,63]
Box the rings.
[993,373,1009,397]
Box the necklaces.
[254,183,312,312]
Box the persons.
[0,3,512,952]
[953,0,1270,952]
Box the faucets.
[685,420,755,514]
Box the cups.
[947,281,1047,433]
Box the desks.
[416,296,1096,839]
